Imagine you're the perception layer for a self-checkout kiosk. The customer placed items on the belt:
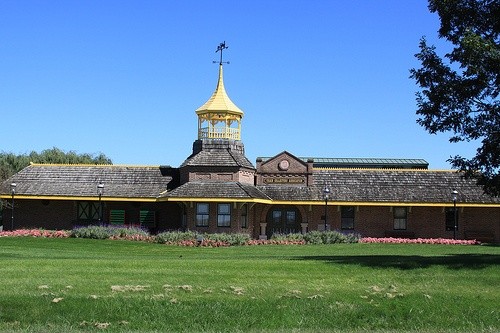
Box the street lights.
[323,187,331,231]
[452,190,459,240]
[97,181,104,226]
[10,181,16,232]
